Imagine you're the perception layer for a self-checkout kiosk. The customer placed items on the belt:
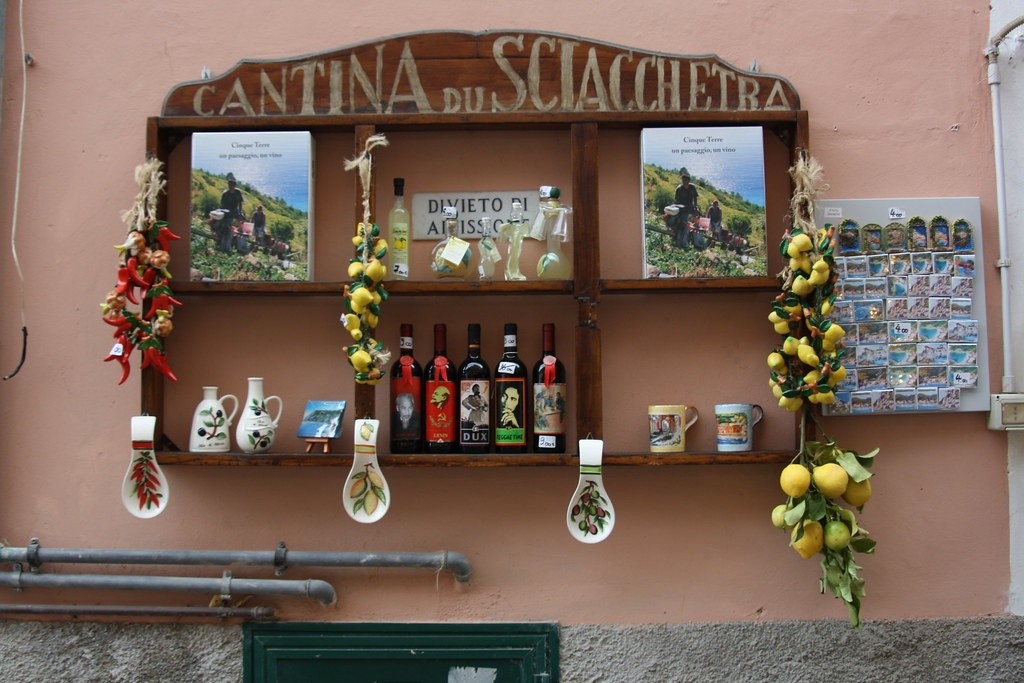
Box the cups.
[648,404,699,453]
[715,403,764,452]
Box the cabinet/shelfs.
[141,28,812,469]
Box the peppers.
[100,226,179,385]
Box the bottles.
[503,202,527,281]
[476,216,496,282]
[457,323,495,454]
[236,377,283,454]
[189,386,238,453]
[431,207,475,282]
[493,322,529,454]
[386,177,410,282]
[533,322,567,455]
[423,323,458,456]
[535,187,573,280]
[389,323,424,454]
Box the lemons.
[768,229,846,412]
[772,463,872,561]
[343,236,390,385]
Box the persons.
[663,174,723,249]
[208,179,267,255]
